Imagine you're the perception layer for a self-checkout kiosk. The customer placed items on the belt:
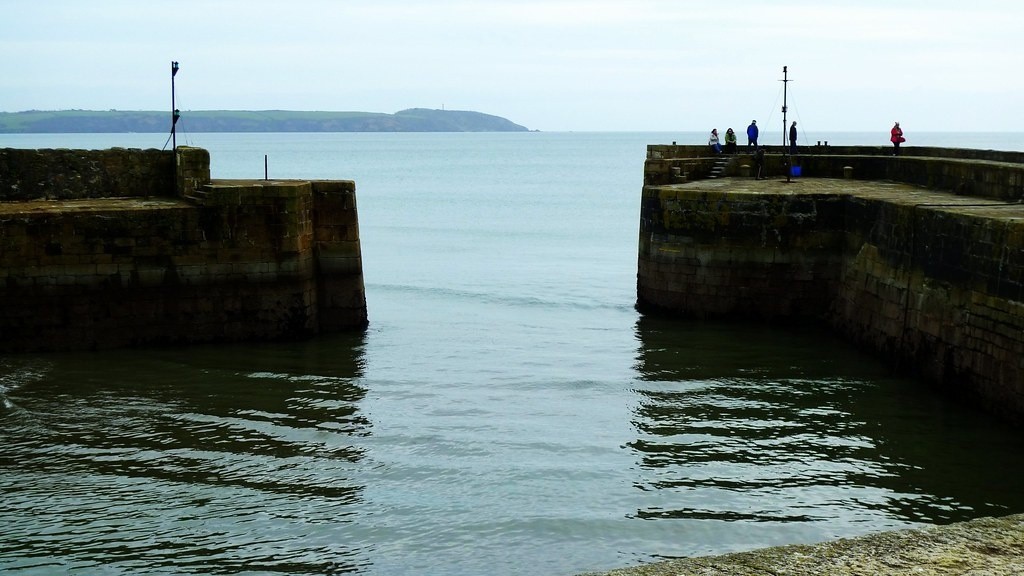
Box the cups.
[818,141,820,146]
[673,142,676,145]
[825,141,827,145]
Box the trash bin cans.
[671,167,681,183]
[843,166,853,179]
[790,166,801,176]
[741,164,750,177]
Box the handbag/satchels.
[897,137,905,142]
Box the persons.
[725,128,737,153]
[710,129,723,154]
[790,121,799,154]
[891,122,903,156]
[747,120,758,154]
[756,149,765,180]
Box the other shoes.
[720,151,722,153]
